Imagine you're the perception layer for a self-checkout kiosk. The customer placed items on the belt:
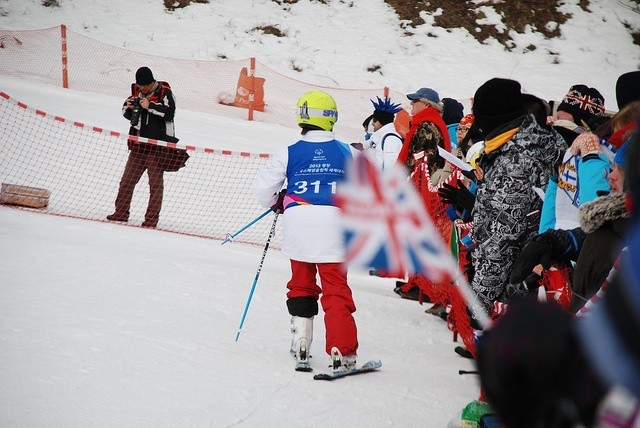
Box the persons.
[106,66,176,227]
[254,90,360,374]
[446,70,639,426]
[398,88,447,298]
[364,102,404,276]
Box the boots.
[289,316,313,358]
[328,354,357,374]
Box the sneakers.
[107,212,129,222]
[142,219,158,227]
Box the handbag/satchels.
[158,145,189,172]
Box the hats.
[472,77,522,131]
[556,85,606,126]
[407,88,440,104]
[374,109,394,125]
[460,114,474,130]
[136,67,154,86]
[613,135,632,167]
[442,98,462,124]
[363,115,373,128]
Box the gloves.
[510,228,564,285]
[438,179,475,211]
[271,192,287,214]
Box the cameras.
[129,96,142,126]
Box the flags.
[332,155,451,274]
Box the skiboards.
[290,351,382,380]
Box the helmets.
[296,90,338,131]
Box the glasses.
[373,118,377,122]
[412,100,419,103]
[455,127,468,132]
